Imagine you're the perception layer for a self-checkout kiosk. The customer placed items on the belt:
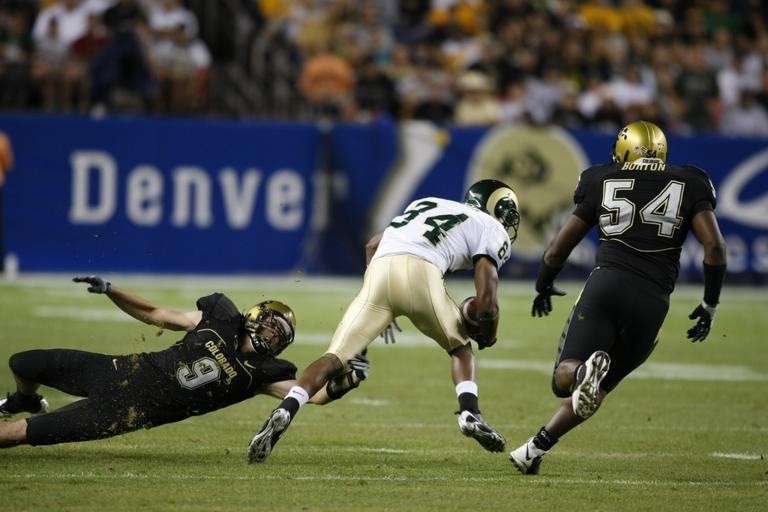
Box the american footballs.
[459,296,479,340]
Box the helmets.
[244,299,297,356]
[612,122,667,162]
[464,180,520,243]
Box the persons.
[247,179,521,464]
[0,0,768,130]
[509,120,728,475]
[0,275,370,448]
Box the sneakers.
[572,349,612,420]
[509,443,542,475]
[248,408,290,467]
[0,394,49,417]
[456,411,507,451]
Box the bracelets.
[326,370,359,399]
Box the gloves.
[532,286,567,320]
[347,347,369,381]
[71,275,110,294]
[379,320,403,345]
[686,303,717,344]
